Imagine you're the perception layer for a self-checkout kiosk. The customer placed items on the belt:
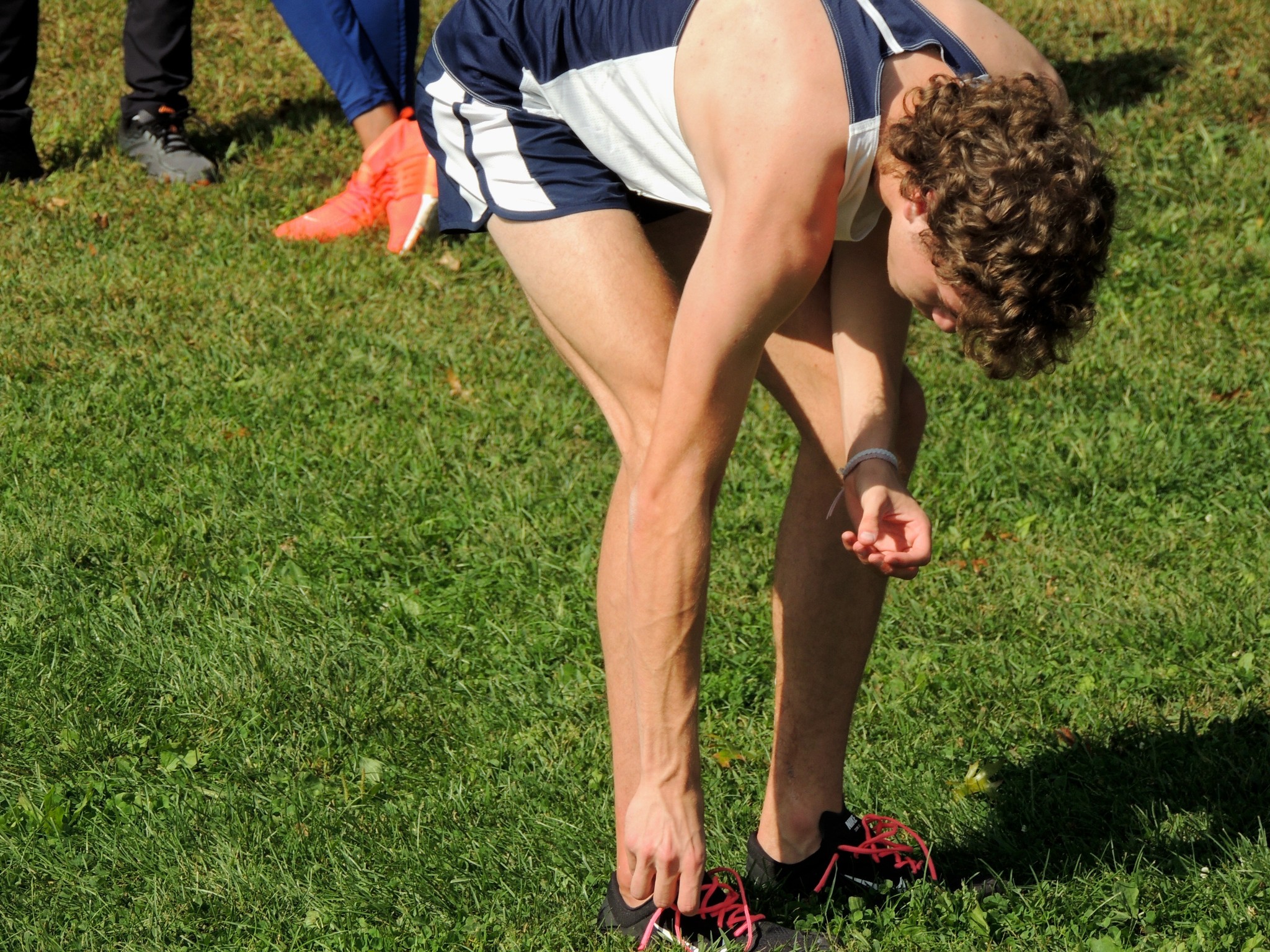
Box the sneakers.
[362,105,440,255]
[745,807,960,926]
[596,861,829,952]
[120,107,216,188]
[269,159,385,240]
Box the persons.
[269,0,440,256]
[1,1,222,188]
[414,0,1121,952]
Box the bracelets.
[826,448,898,520]
